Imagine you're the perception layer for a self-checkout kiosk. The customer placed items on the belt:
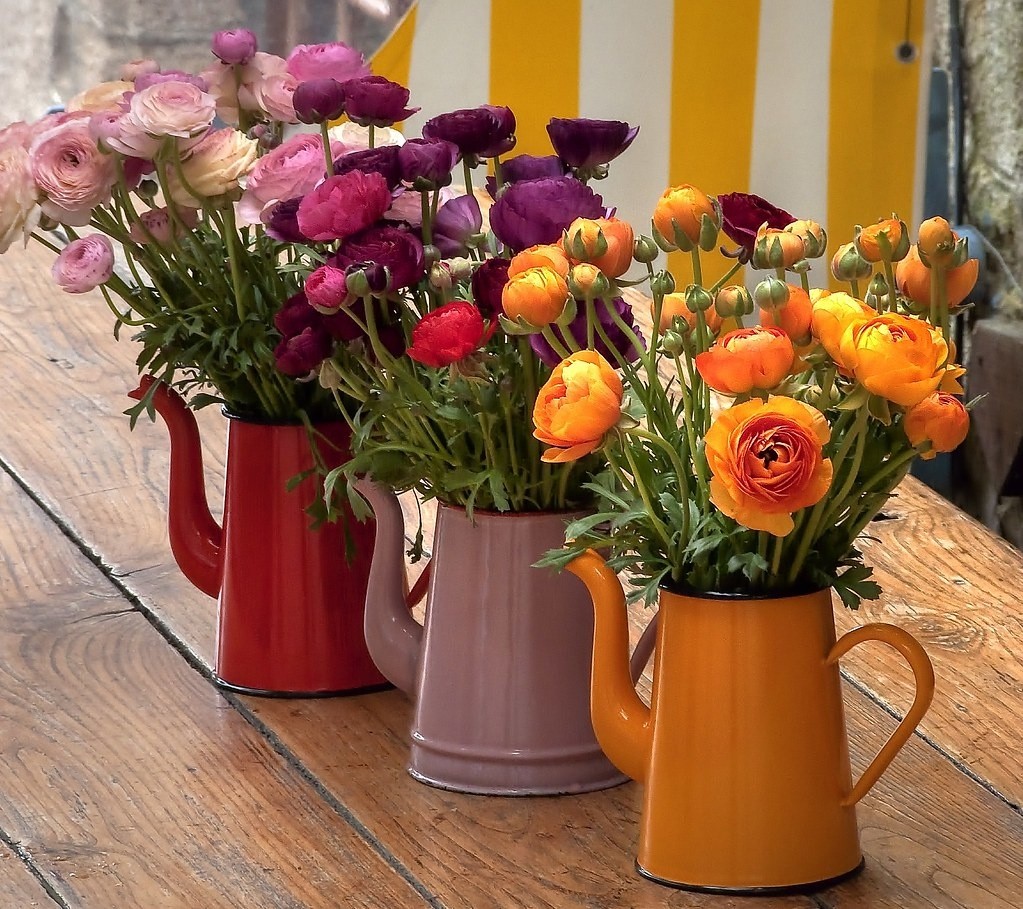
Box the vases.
[343,471,635,797]
[126,374,435,696]
[556,555,934,895]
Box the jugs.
[125,367,441,697]
[337,470,663,800]
[559,538,935,894]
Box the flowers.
[0,32,980,539]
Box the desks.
[0,186,1022,909]
